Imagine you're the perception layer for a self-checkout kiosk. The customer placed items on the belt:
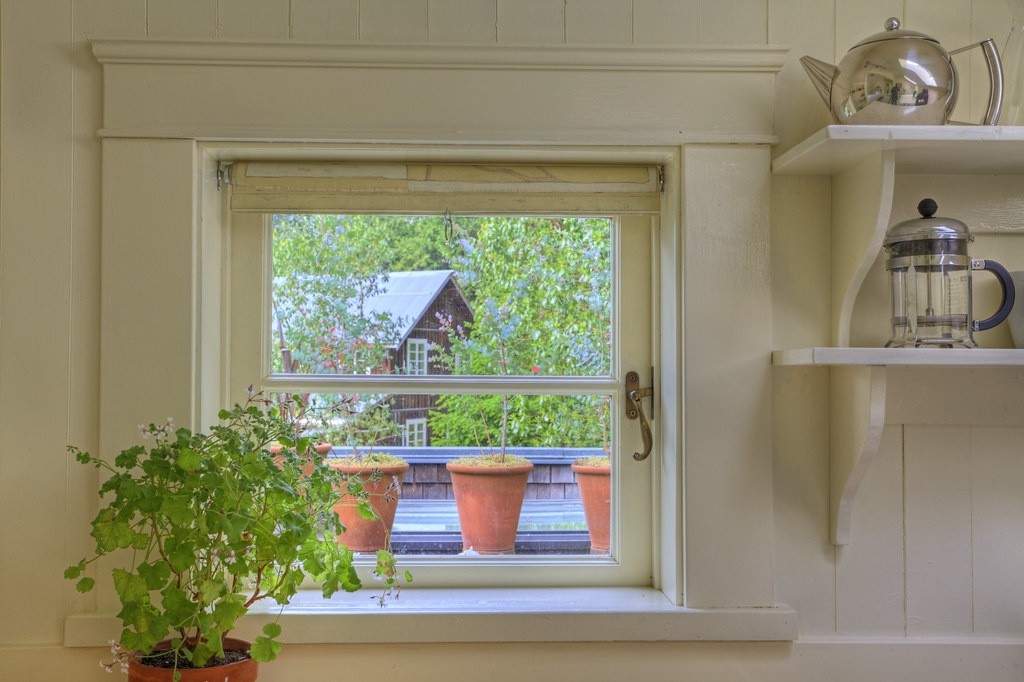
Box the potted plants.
[270,349,335,517]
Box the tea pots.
[800,17,1003,126]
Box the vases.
[572,457,611,553]
[128,636,259,682]
[444,455,533,551]
[325,456,411,551]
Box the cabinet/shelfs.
[769,120,1024,545]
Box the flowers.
[271,213,407,464]
[434,232,548,464]
[58,382,421,682]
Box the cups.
[883,199,1016,349]
